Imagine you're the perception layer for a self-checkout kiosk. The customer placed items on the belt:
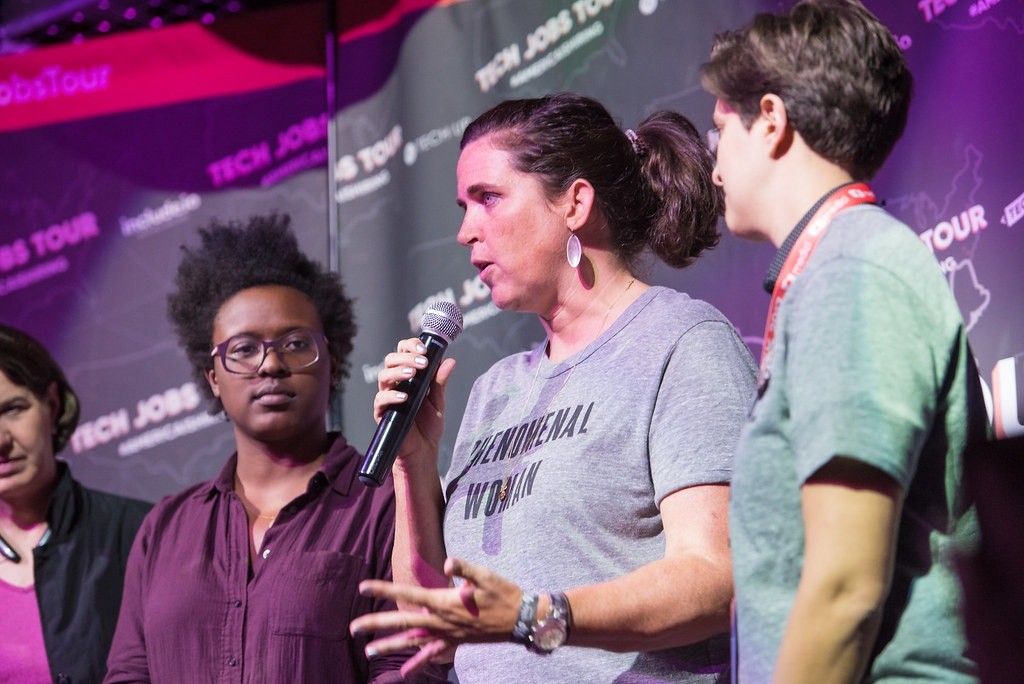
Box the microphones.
[355,302,463,489]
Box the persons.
[103,212,448,684]
[0,325,152,684]
[348,91,760,684]
[696,1,991,684]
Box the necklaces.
[233,475,275,529]
[499,276,635,501]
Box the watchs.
[525,591,567,655]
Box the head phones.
[761,182,853,296]
[0,530,52,564]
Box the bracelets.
[511,590,539,643]
[561,592,572,629]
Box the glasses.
[209,328,329,377]
